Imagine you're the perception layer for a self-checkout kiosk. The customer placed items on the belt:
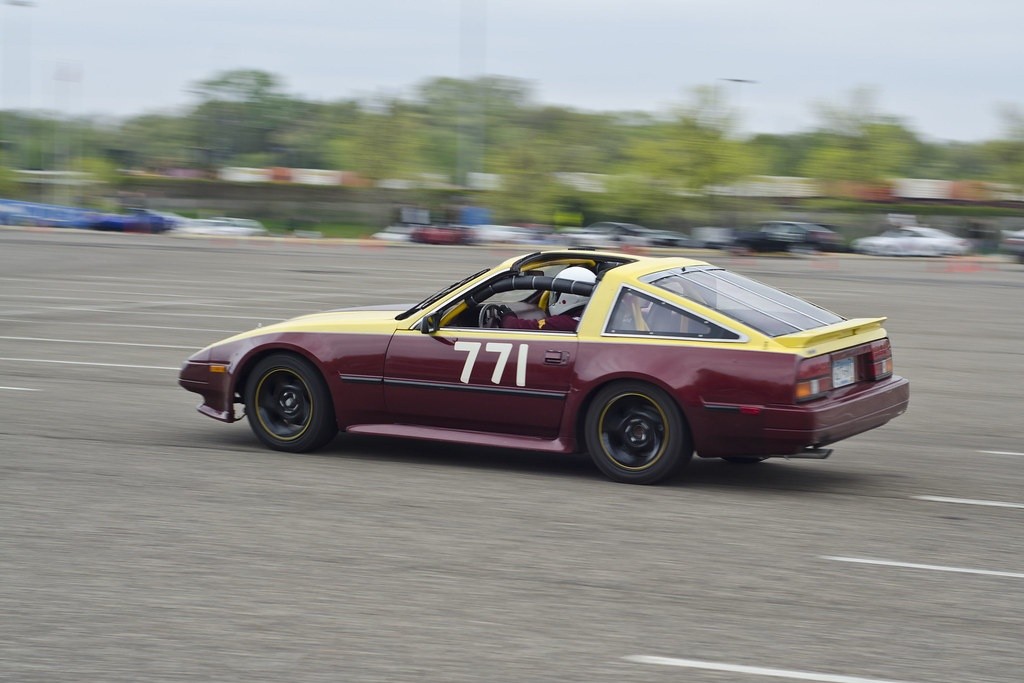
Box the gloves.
[498,304,513,319]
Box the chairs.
[611,302,625,330]
[645,302,672,331]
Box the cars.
[178,246,910,487]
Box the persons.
[495,267,598,332]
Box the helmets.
[548,266,597,316]
